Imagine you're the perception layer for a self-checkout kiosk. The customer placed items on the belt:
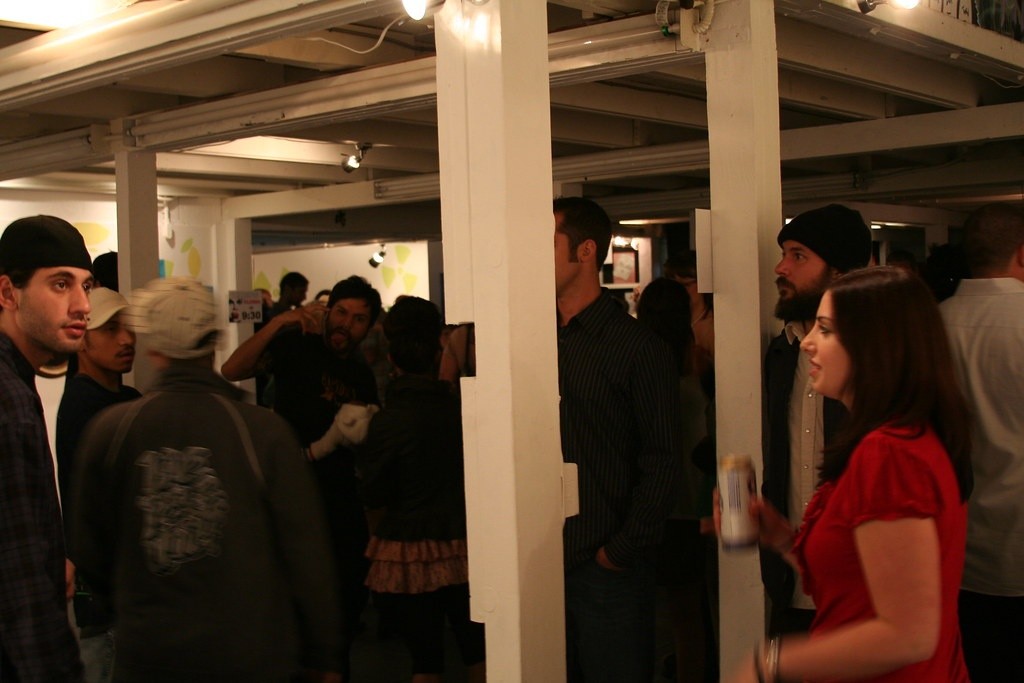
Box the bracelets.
[753,634,781,683]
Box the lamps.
[341,142,372,173]
[857,0,919,14]
[401,0,445,21]
[368,242,387,269]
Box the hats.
[0,215,94,278]
[86,287,129,331]
[132,277,225,358]
[777,204,872,276]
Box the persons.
[554,196,720,683]
[761,204,871,639]
[34,253,143,682]
[221,273,487,683]
[938,205,1024,683]
[0,215,92,683]
[74,281,343,683]
[714,269,973,683]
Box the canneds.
[717,453,759,546]
